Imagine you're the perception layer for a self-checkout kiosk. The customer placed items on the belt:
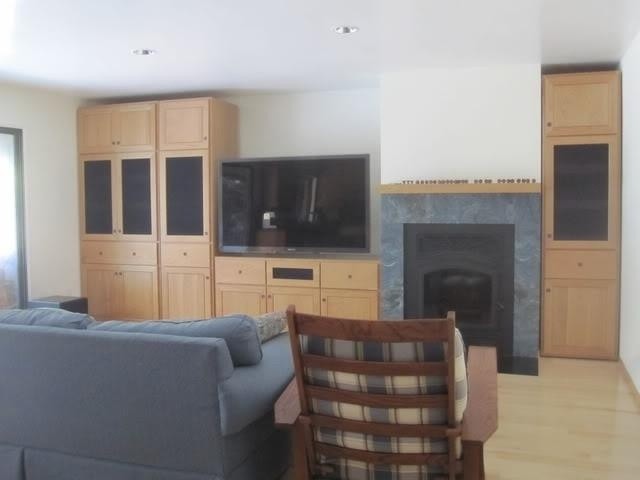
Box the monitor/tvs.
[216,153,371,254]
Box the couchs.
[0,306,296,480]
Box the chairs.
[273,304,499,480]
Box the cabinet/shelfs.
[214,251,382,334]
[76,101,160,322]
[541,70,623,360]
[156,96,240,321]
